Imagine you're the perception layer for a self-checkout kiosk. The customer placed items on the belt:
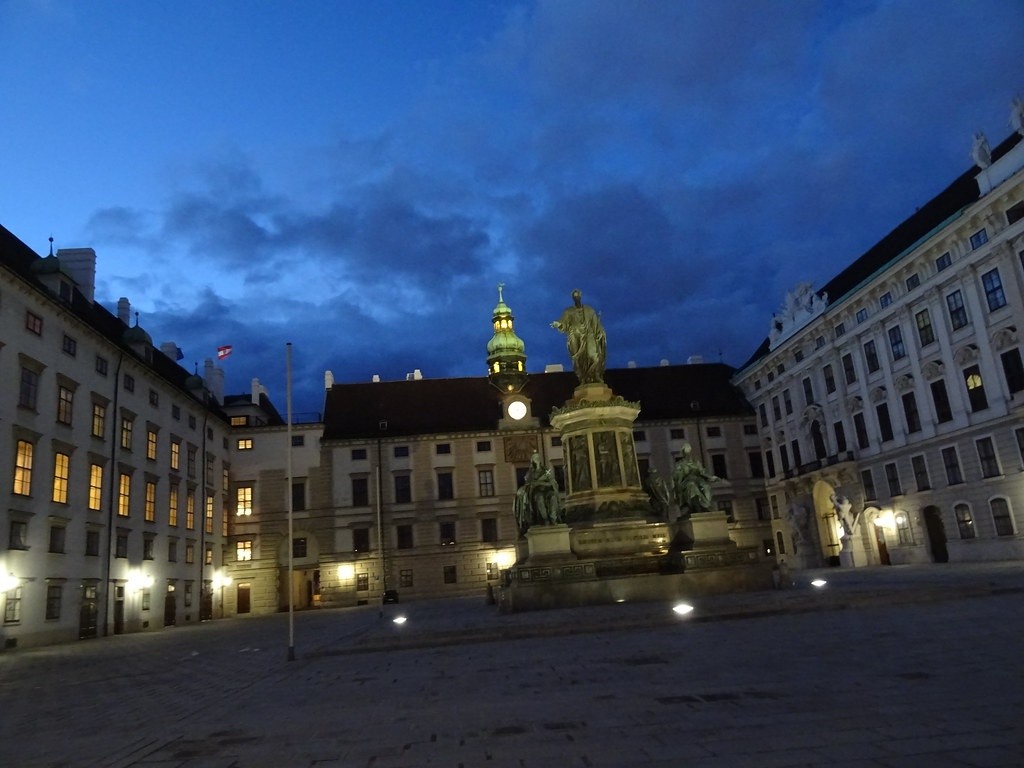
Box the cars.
[382,590,398,606]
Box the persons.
[779,559,789,588]
[572,437,590,490]
[831,494,853,532]
[549,289,606,385]
[1006,99,1024,130]
[971,132,992,170]
[524,454,561,526]
[599,434,615,487]
[670,443,718,512]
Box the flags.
[217,345,232,360]
